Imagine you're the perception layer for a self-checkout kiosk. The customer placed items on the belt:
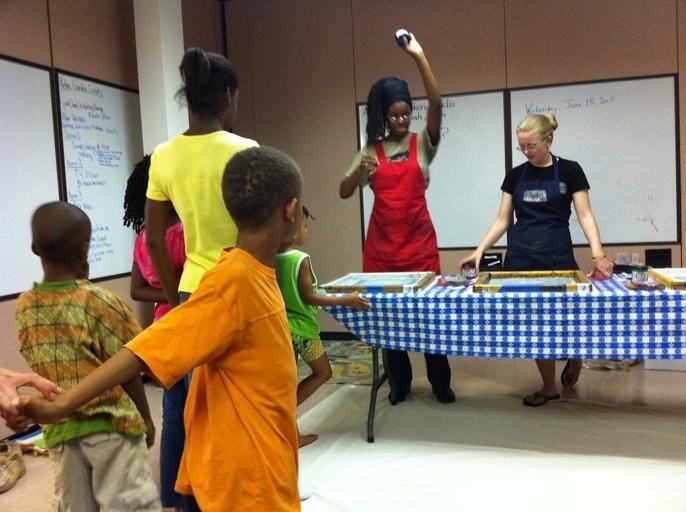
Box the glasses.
[516,136,547,150]
[385,112,412,121]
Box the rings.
[365,162,368,167]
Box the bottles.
[394,28,411,47]
[461,262,477,280]
[631,264,649,284]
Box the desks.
[315,275,686,446]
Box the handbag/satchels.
[0,439,25,494]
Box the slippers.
[522,391,560,407]
[560,359,582,388]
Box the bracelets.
[591,254,608,262]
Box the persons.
[0,363,65,434]
[144,47,262,511]
[121,154,194,511]
[5,146,305,511]
[337,28,458,407]
[273,205,371,447]
[12,199,163,512]
[457,114,616,407]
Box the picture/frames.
[650,265,686,288]
[324,271,436,294]
[472,269,593,293]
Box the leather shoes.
[389,383,411,403]
[431,384,455,403]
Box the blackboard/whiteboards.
[355,90,509,248]
[509,74,679,248]
[0,52,143,303]
